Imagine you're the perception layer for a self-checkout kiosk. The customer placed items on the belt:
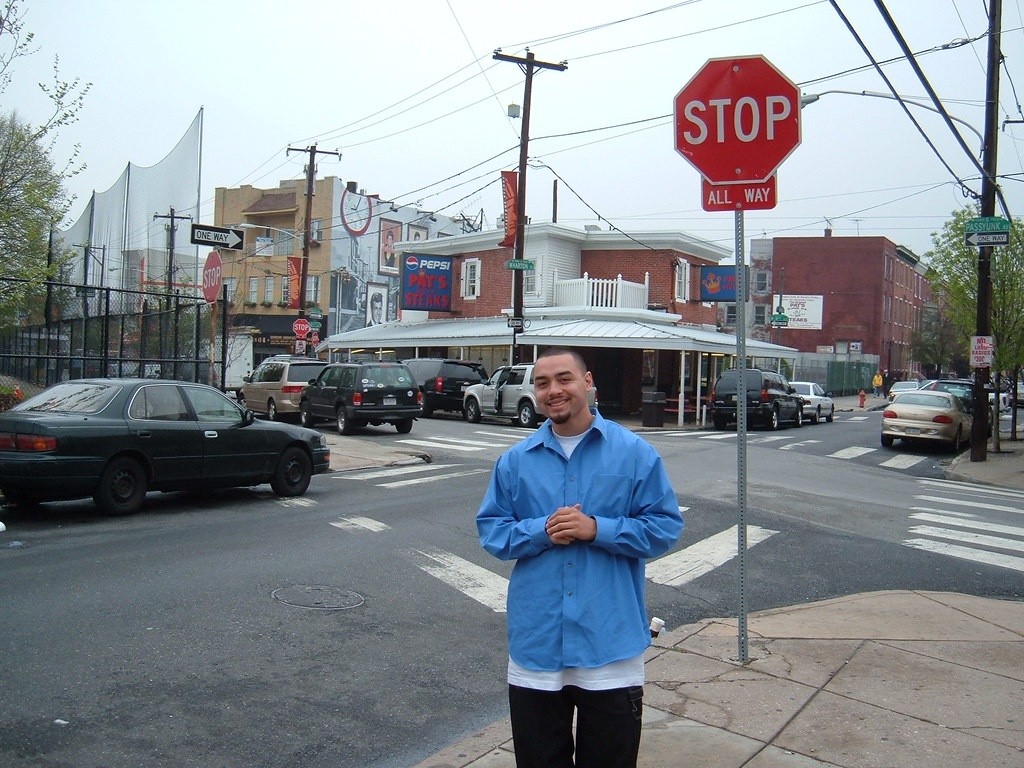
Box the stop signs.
[202,250,223,304]
[293,319,311,338]
[672,53,802,185]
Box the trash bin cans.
[641,391,667,426]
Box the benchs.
[664,404,707,424]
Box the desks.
[690,396,710,417]
[665,398,692,424]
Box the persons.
[873,371,882,398]
[477,348,684,767]
[385,230,395,267]
[883,369,892,399]
[368,293,383,326]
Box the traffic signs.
[506,317,523,329]
[963,230,1012,247]
[188,224,246,252]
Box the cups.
[648,617,665,639]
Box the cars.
[788,381,836,423]
[889,379,1024,437]
[879,390,973,453]
[1,378,331,513]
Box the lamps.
[377,201,398,212]
[455,217,472,228]
[417,211,436,223]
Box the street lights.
[241,224,310,318]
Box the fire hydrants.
[859,390,865,407]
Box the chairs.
[908,398,920,404]
[336,373,354,387]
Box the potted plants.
[245,302,256,308]
[278,301,286,307]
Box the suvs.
[299,360,423,435]
[710,367,803,431]
[462,363,598,428]
[402,358,490,418]
[239,354,330,420]
